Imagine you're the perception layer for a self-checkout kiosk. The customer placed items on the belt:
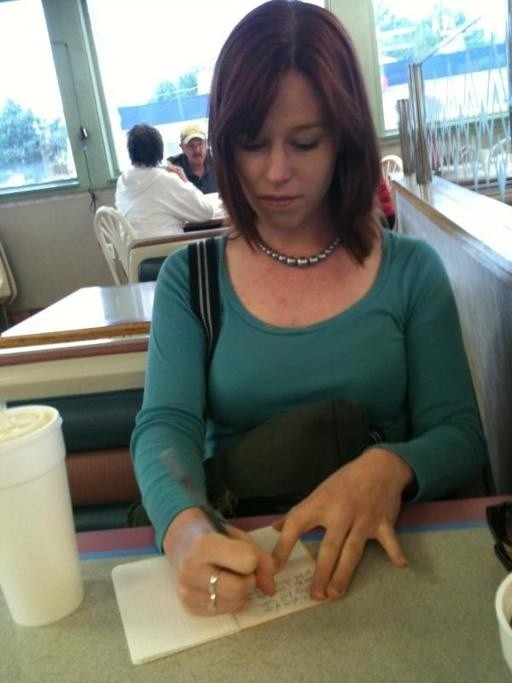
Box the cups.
[0,405,85,629]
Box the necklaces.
[255,238,342,266]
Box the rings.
[208,594,216,613]
[208,569,220,594]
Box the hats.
[179,126,207,145]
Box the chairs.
[93,205,137,286]
[375,153,403,234]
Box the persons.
[167,124,219,194]
[129,0,491,616]
[115,123,214,282]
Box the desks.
[1,491,511,682]
[2,279,158,414]
[129,225,231,287]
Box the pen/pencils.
[162,448,230,536]
